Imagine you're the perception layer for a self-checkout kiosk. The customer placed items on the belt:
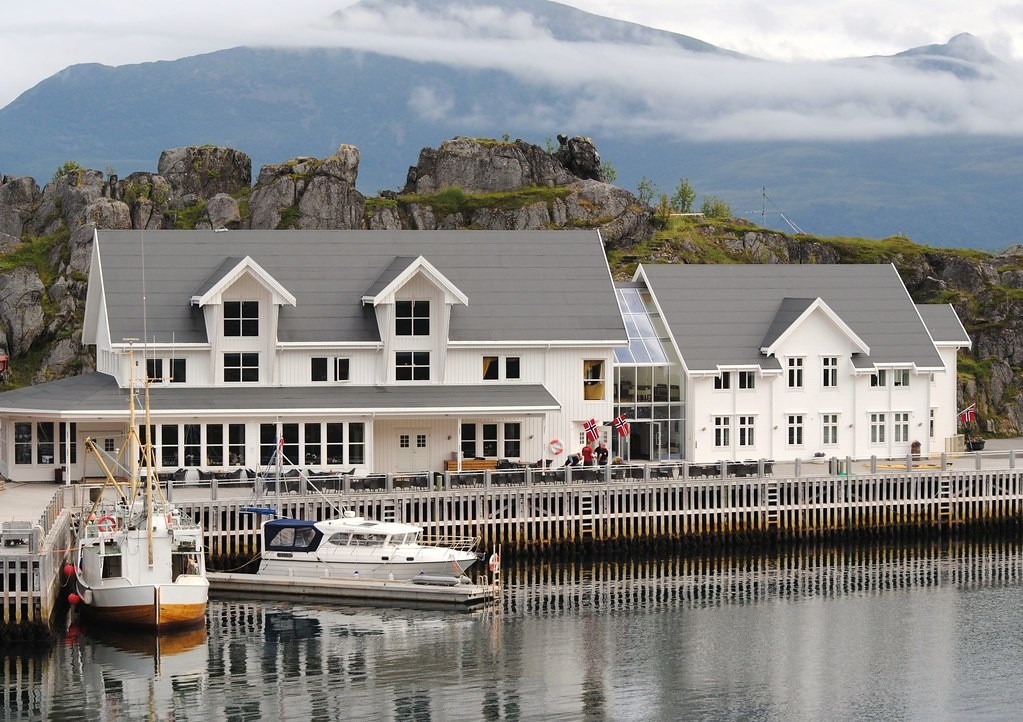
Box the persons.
[582,442,594,466]
[564,452,583,466]
[594,441,608,465]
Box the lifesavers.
[549,439,564,454]
[98,516,117,533]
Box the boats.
[209,416,503,612]
[69,226,209,631]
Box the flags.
[583,419,598,442]
[614,415,629,437]
[962,406,975,422]
